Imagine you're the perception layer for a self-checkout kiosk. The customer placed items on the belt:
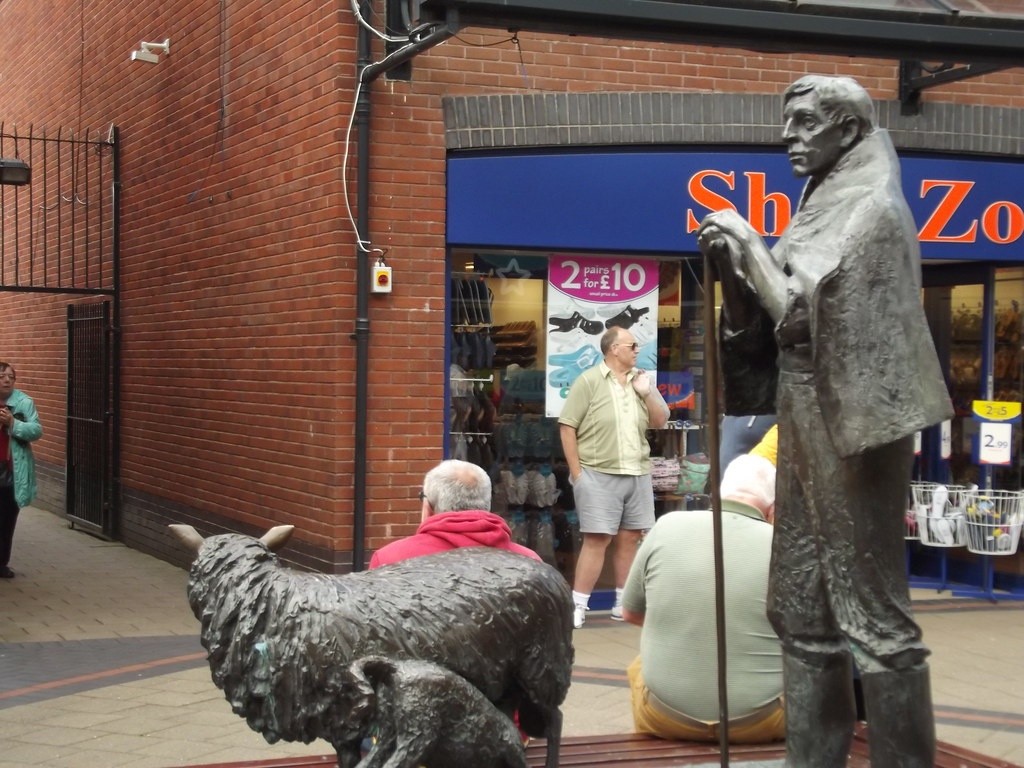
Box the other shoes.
[572,604,590,630]
[610,605,625,621]
[531,423,553,458]
[0,566,16,578]
[451,393,497,433]
[504,424,528,458]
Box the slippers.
[549,345,604,367]
[451,278,494,324]
[549,353,603,387]
[451,334,497,368]
[635,339,657,371]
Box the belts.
[645,691,780,734]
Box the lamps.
[0,157,31,186]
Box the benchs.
[179,720,1024,768]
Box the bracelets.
[642,390,652,401]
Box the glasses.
[419,490,436,511]
[623,342,639,351]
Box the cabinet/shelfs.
[447,270,495,437]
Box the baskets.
[960,488,1024,556]
[910,483,978,549]
[903,482,924,541]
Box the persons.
[620,452,790,746]
[367,455,547,569]
[715,411,779,469]
[559,324,671,629]
[0,360,44,578]
[696,73,956,768]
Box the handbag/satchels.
[673,453,711,494]
[648,456,680,492]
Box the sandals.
[517,473,529,505]
[502,472,516,503]
[547,473,562,506]
[605,306,650,329]
[533,474,548,507]
[549,311,603,335]
